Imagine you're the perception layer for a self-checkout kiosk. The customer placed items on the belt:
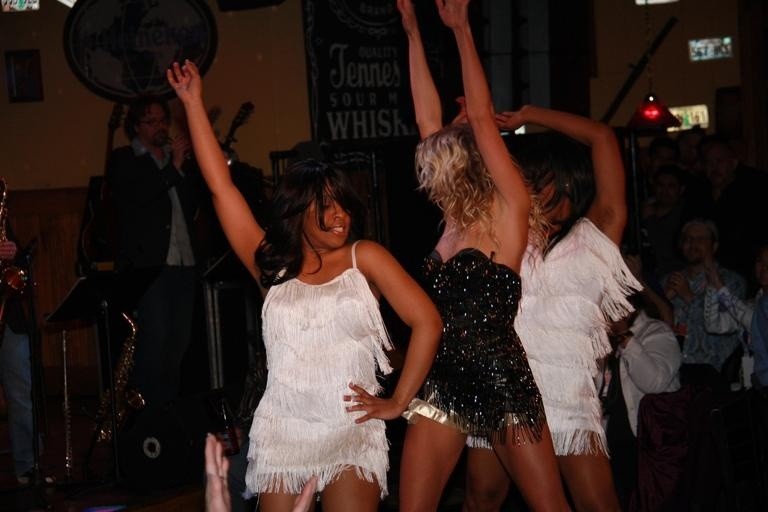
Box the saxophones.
[88,311,146,448]
[0,173,28,328]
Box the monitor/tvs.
[667,103,710,136]
[686,34,735,63]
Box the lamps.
[626,0,682,127]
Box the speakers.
[111,398,203,501]
[5,48,45,103]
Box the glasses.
[139,117,167,125]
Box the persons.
[394,0,570,512]
[166,58,446,512]
[201,433,318,512]
[0,201,59,498]
[452,91,647,510]
[597,127,768,512]
[104,95,247,449]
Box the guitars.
[223,102,254,147]
[79,102,126,263]
